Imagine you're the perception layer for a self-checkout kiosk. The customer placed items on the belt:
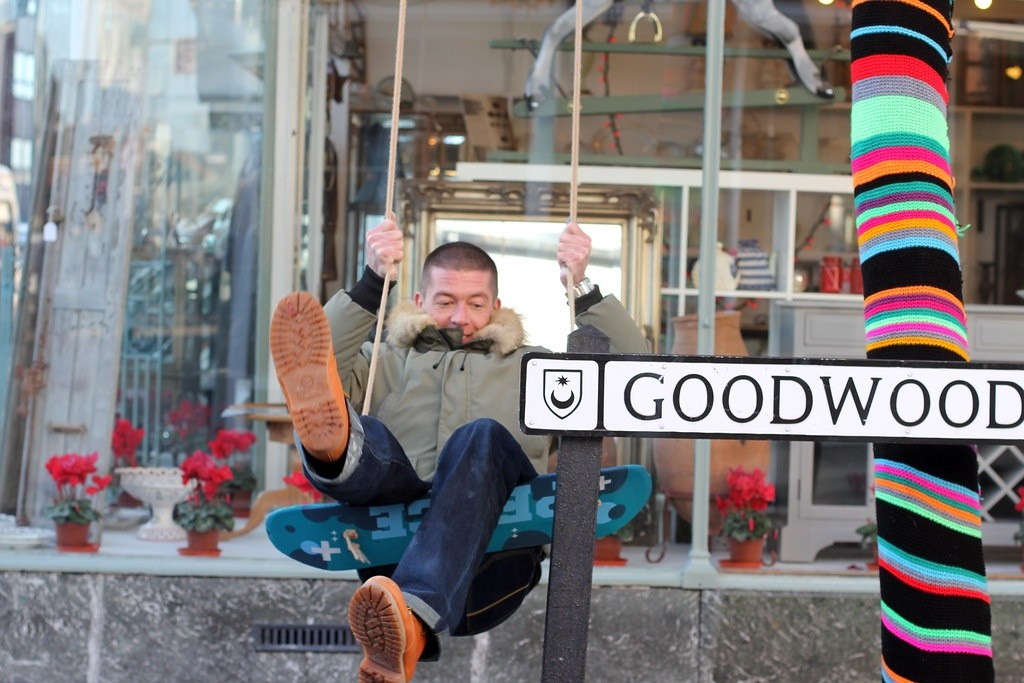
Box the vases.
[178,528,222,557]
[230,487,253,519]
[719,534,765,570]
[866,544,877,572]
[54,520,101,553]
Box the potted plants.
[592,507,645,567]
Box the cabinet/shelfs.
[818,102,1023,302]
[455,159,863,316]
[774,300,1024,564]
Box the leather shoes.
[270,290,350,461]
[349,575,426,683]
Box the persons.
[266,205,652,683]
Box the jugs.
[690,241,741,291]
[730,239,777,291]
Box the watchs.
[564,277,596,302]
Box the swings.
[263,1,654,573]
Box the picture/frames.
[396,176,658,544]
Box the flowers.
[170,448,237,533]
[207,428,257,493]
[1013,485,1024,544]
[44,452,113,525]
[282,468,338,504]
[854,483,877,551]
[116,385,212,468]
[111,416,145,473]
[714,464,776,546]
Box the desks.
[221,404,322,539]
